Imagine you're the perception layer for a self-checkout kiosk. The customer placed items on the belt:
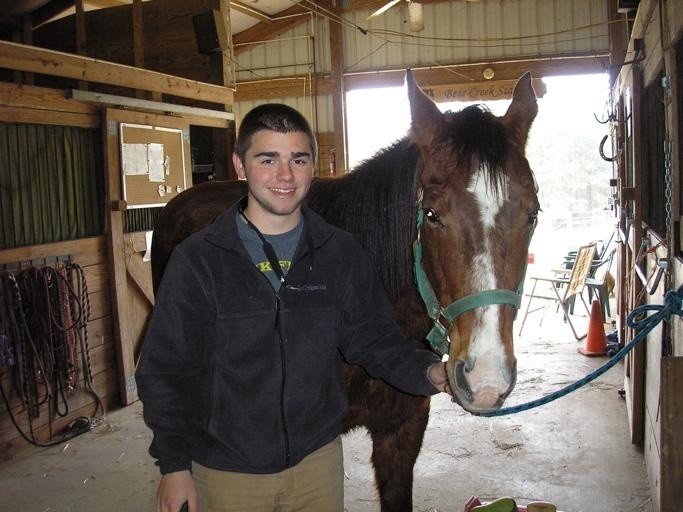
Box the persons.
[136,104,454,512]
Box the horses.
[150,69,543,512]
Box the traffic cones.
[578,300,607,356]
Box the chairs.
[519,232,617,340]
